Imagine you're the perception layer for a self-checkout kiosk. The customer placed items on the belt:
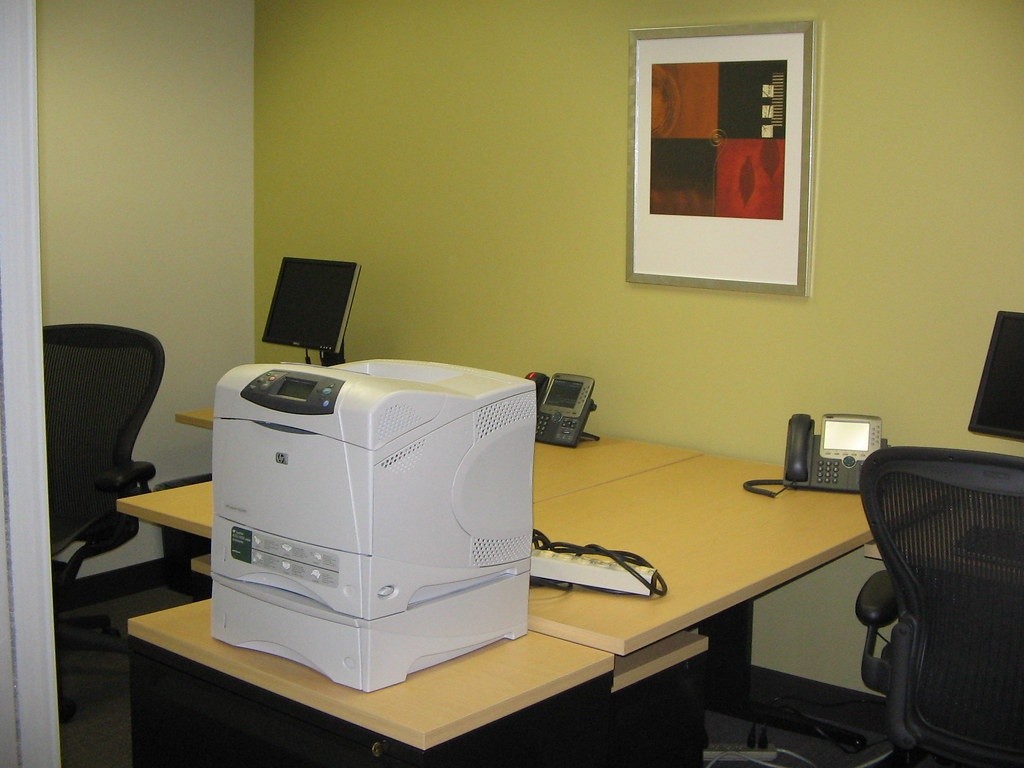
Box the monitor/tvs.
[967,311,1024,442]
[261,257,362,367]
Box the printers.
[210,359,537,693]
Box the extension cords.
[702,742,777,761]
[530,548,659,596]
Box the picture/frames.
[625,19,817,298]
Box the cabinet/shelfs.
[610,630,709,768]
[189,553,215,603]
[128,599,615,768]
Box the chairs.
[855,447,1024,768]
[43,323,167,726]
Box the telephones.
[525,370,596,449]
[782,413,884,493]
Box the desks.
[519,454,874,768]
[174,406,215,433]
[115,440,701,544]
